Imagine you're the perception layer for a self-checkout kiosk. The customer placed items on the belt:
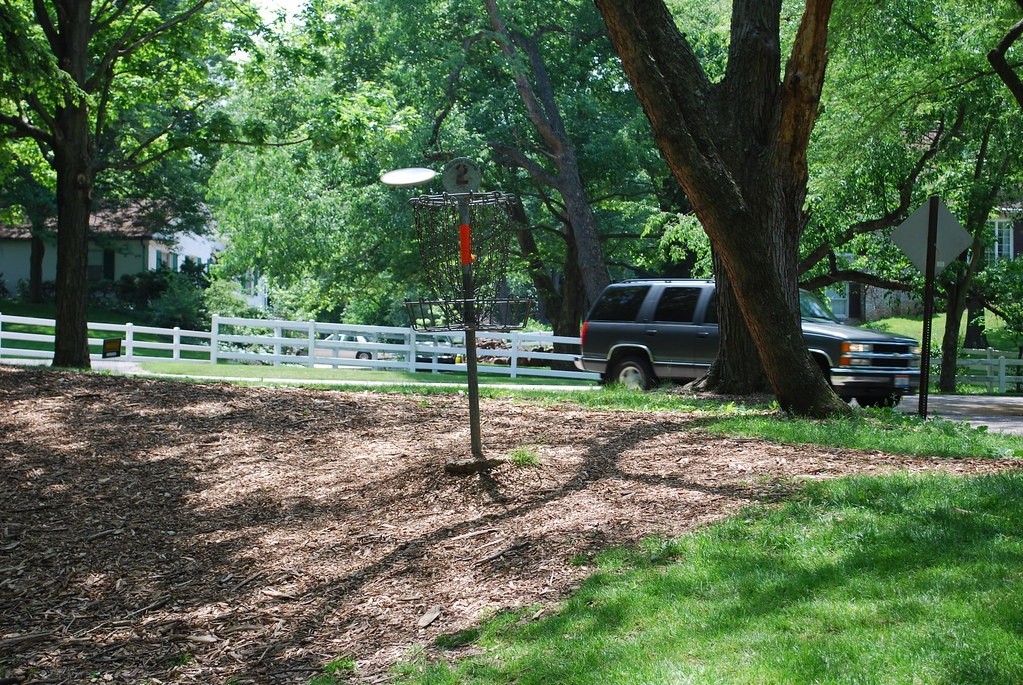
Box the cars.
[415,336,454,363]
[297,333,400,361]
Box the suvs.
[575,279,921,408]
